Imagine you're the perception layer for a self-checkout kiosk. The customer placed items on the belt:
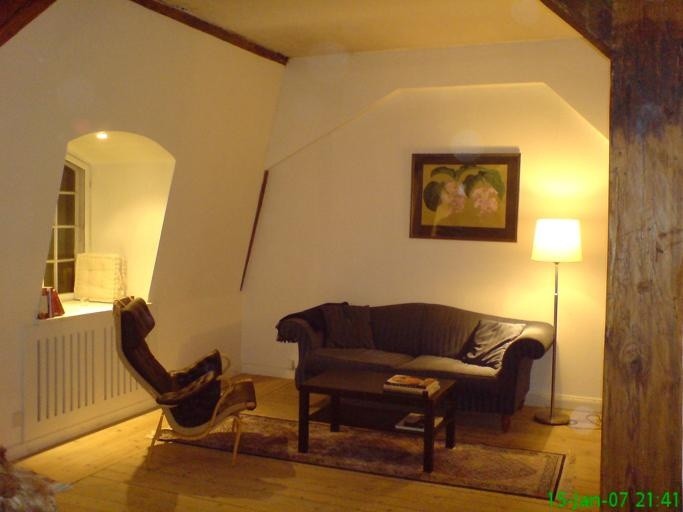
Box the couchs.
[275,298,553,436]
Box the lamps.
[526,216,585,426]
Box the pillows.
[69,252,129,307]
[457,319,525,370]
[321,301,378,351]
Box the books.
[394,411,443,432]
[36,287,52,318]
[49,289,64,316]
[382,383,440,399]
[384,374,436,389]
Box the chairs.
[111,293,261,469]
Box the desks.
[295,371,457,476]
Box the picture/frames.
[407,151,522,245]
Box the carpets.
[150,408,565,502]
